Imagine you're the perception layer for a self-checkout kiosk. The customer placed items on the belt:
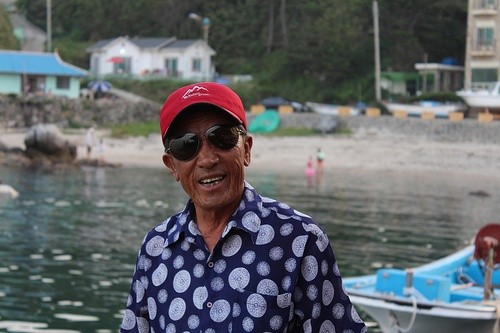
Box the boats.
[342,240,500,333]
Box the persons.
[97,140,105,165]
[305,156,315,186]
[118,82,372,333]
[316,148,324,176]
[85,124,97,161]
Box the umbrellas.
[87,80,114,93]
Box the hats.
[159,81,248,141]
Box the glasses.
[162,125,247,162]
